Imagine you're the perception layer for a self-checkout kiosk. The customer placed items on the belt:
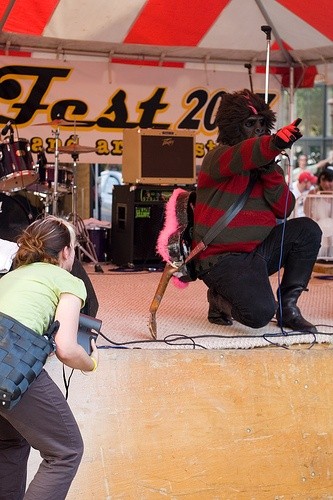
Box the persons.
[0,211,99,500]
[285,151,333,257]
[192,88,323,334]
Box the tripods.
[65,176,100,261]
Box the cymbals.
[48,145,97,154]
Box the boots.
[276,284,317,333]
[206,289,233,326]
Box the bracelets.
[81,357,97,376]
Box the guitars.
[147,190,198,340]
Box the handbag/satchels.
[0,312,61,410]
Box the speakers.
[122,129,197,186]
[109,183,187,269]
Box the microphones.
[1,120,12,136]
[280,150,288,157]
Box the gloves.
[274,117,303,150]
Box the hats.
[298,172,318,184]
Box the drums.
[0,138,40,192]
[76,219,112,265]
[26,164,74,195]
[0,191,38,243]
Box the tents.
[1,0,333,191]
